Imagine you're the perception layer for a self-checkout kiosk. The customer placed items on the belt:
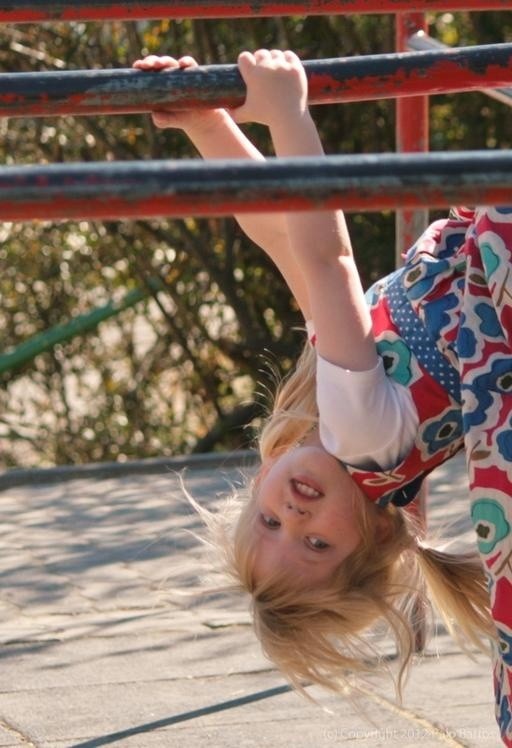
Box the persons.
[131,46,512,747]
